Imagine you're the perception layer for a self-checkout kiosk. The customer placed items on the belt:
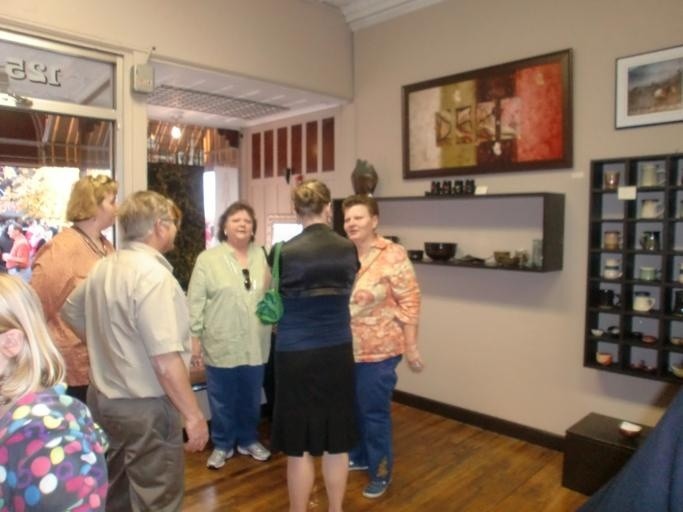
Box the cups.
[384,236,422,261]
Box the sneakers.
[236,440,272,462]
[204,448,237,470]
[361,471,397,499]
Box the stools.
[562,413,657,498]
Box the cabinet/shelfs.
[582,153,683,384]
[332,192,565,273]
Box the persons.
[263,179,361,512]
[60,191,210,512]
[186,202,273,470]
[28,174,118,406]
[0,273,111,512]
[0,214,57,284]
[341,195,426,499]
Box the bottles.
[431,178,475,195]
[591,163,683,378]
[494,239,543,270]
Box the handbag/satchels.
[253,286,285,326]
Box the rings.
[415,363,420,368]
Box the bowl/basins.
[424,241,456,262]
[619,425,643,436]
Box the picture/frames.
[615,44,683,130]
[401,47,572,178]
